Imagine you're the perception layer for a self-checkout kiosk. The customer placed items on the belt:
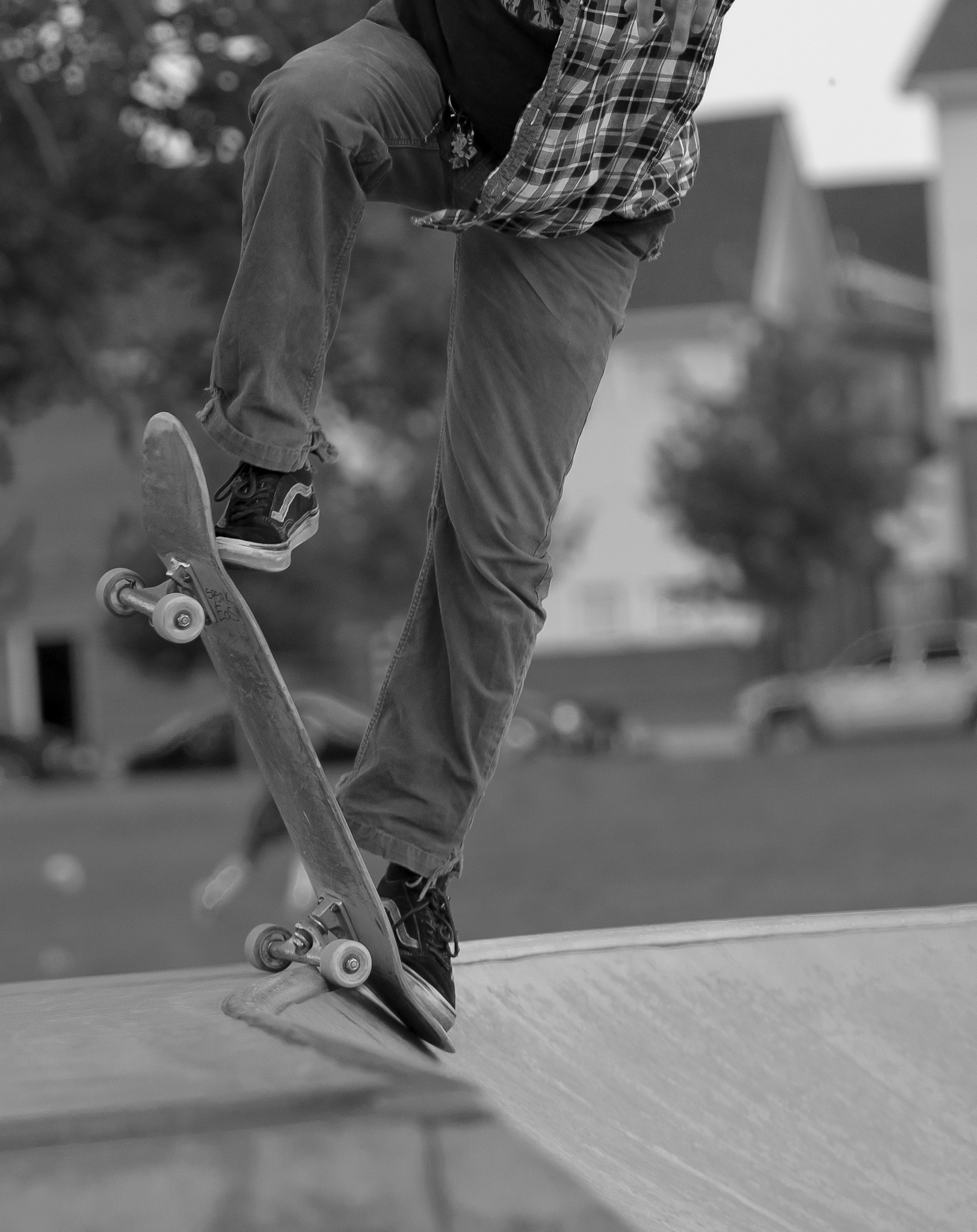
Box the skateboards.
[95,406,461,1055]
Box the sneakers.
[214,461,321,571]
[375,875,460,1031]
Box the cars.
[738,617,974,750]
[125,693,371,777]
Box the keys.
[423,105,476,168]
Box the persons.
[198,0,734,1032]
